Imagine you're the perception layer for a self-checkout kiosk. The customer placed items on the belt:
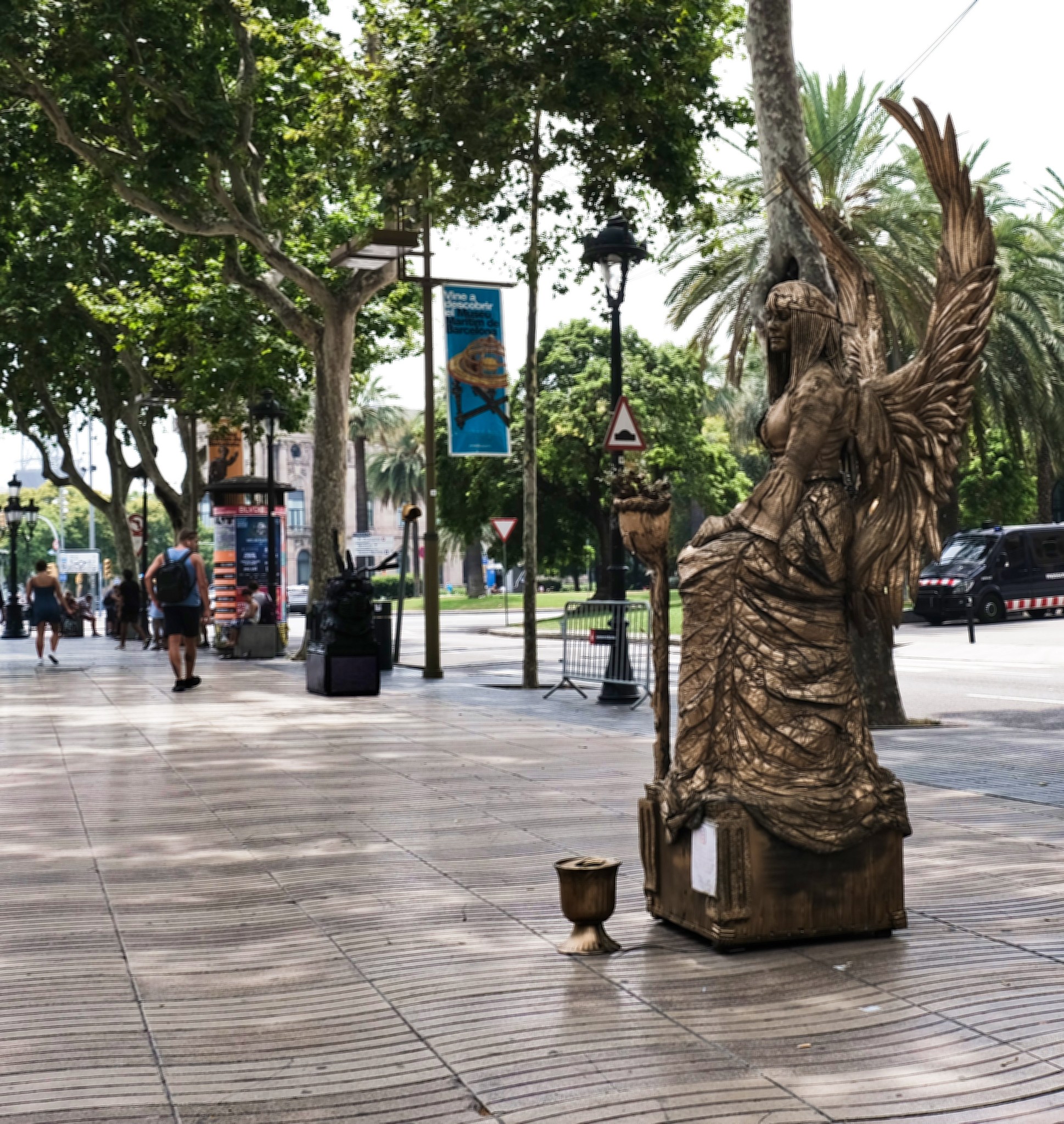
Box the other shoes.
[173,676,202,692]
[219,654,231,659]
[93,633,99,636]
[47,650,60,665]
[35,658,44,668]
[141,638,151,650]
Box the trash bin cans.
[372,600,394,672]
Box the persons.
[0,558,276,666]
[655,278,913,852]
[145,527,210,693]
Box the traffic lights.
[402,503,423,523]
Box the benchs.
[105,615,142,640]
[232,621,278,659]
[59,618,85,639]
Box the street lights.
[0,473,40,640]
[252,395,287,657]
[578,216,654,706]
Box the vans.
[913,518,1064,627]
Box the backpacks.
[259,593,277,624]
[156,549,198,607]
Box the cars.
[287,584,309,615]
